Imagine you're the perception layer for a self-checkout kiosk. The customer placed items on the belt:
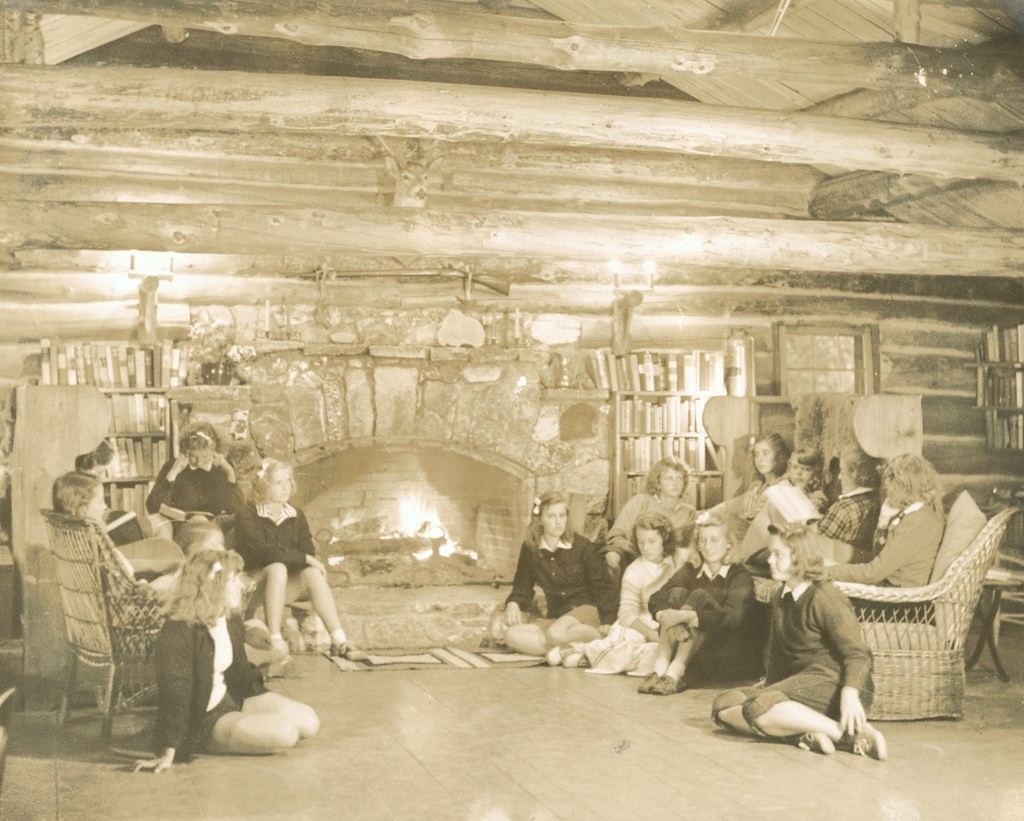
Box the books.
[158,503,214,522]
[986,567,1024,581]
[40,339,181,515]
[583,342,746,511]
[767,485,824,525]
[974,322,1024,545]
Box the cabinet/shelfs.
[100,387,171,484]
[964,360,1023,499]
[613,347,729,521]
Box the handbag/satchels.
[855,600,937,626]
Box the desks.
[964,570,1024,683]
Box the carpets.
[323,646,547,672]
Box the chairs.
[40,509,167,742]
[835,506,1019,721]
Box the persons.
[605,457,698,572]
[499,491,620,655]
[638,511,765,694]
[181,520,305,681]
[792,448,883,549]
[234,458,369,661]
[708,431,830,546]
[128,551,319,774]
[146,421,244,548]
[545,512,688,676]
[830,452,944,622]
[711,521,887,761]
[52,470,176,592]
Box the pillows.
[928,489,986,586]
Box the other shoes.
[798,733,835,755]
[853,722,886,760]
[545,646,563,666]
[652,676,686,695]
[637,672,659,693]
[563,654,585,668]
[329,642,366,661]
